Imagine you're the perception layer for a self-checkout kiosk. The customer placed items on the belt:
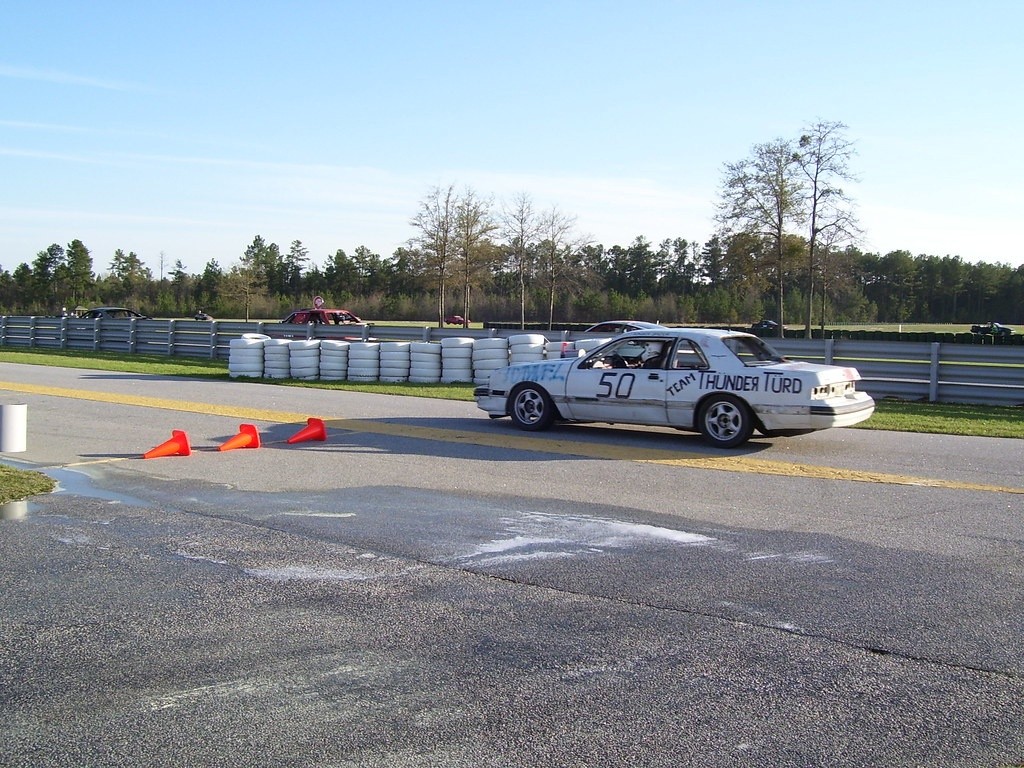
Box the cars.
[265,309,376,343]
[752,321,787,330]
[473,329,875,448]
[55,307,154,344]
[573,321,670,351]
[195,313,214,321]
[446,316,471,325]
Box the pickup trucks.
[971,322,1015,336]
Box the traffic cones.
[287,417,325,443]
[143,430,190,461]
[219,424,260,451]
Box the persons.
[990,321,998,333]
[626,343,662,369]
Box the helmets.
[641,341,664,362]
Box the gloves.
[612,353,623,362]
